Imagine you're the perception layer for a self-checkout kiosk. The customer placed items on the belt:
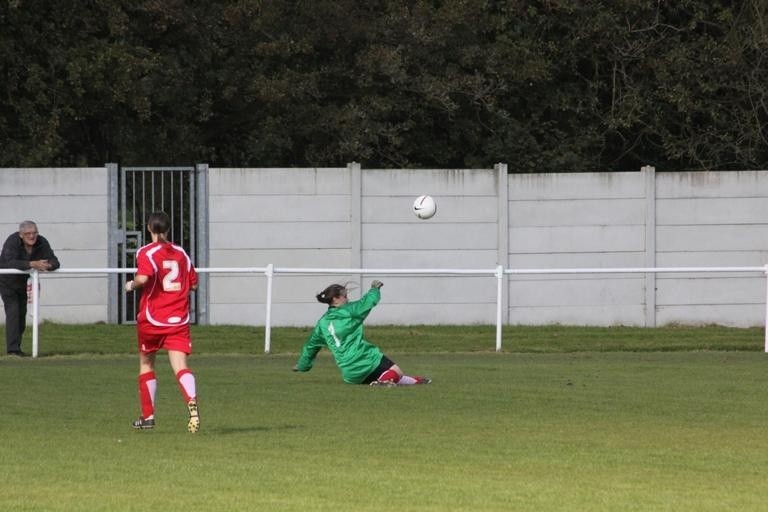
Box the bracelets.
[126,280,133,292]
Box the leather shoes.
[7,350,29,358]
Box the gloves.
[371,280,384,289]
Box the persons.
[124,210,200,433]
[292,279,432,385]
[0,220,61,356]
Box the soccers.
[413,195,436,219]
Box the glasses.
[22,230,38,238]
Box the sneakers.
[186,398,200,434]
[420,379,432,384]
[370,380,397,388]
[132,414,154,429]
